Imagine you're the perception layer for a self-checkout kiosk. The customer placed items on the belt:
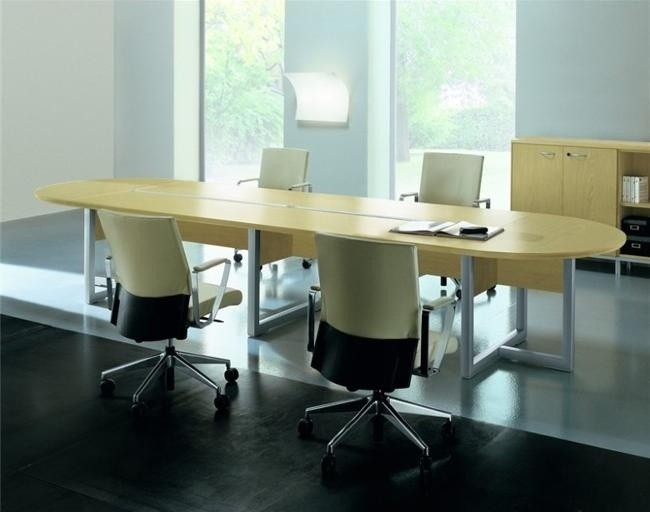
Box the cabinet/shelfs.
[510,137,650,278]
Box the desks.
[34,179,627,380]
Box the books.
[389,216,506,244]
[622,176,650,205]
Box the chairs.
[234,147,322,270]
[399,152,497,301]
[296,230,454,476]
[98,207,240,421]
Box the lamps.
[282,68,352,129]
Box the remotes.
[459,226,488,235]
